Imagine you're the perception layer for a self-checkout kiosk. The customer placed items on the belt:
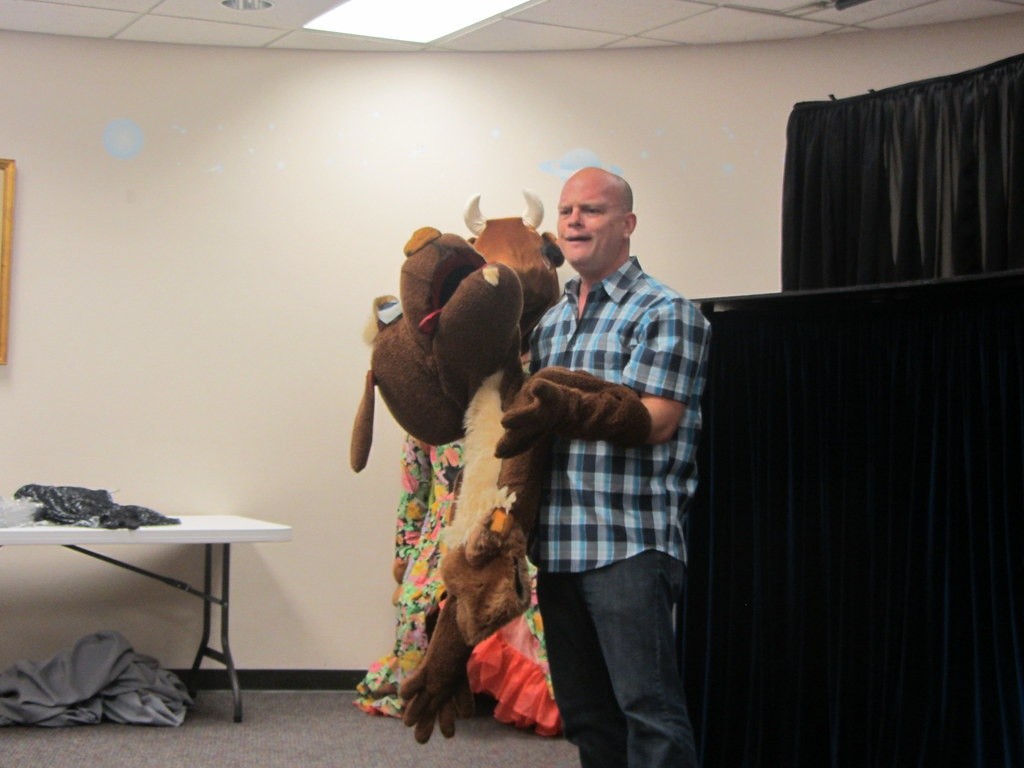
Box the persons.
[496,167,710,768]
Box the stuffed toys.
[349,192,654,747]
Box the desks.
[0,513,292,724]
